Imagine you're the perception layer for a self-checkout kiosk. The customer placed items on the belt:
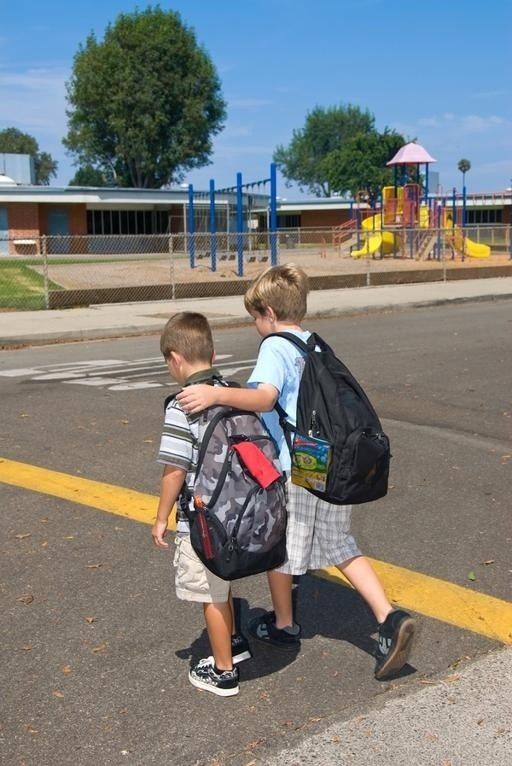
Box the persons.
[176,263,417,681]
[150,316,253,697]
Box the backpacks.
[258,331,391,505]
[164,380,285,579]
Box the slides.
[351,186,490,259]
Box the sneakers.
[231,634,252,664]
[188,657,238,697]
[248,612,302,652]
[375,611,417,679]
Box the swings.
[193,183,268,263]
